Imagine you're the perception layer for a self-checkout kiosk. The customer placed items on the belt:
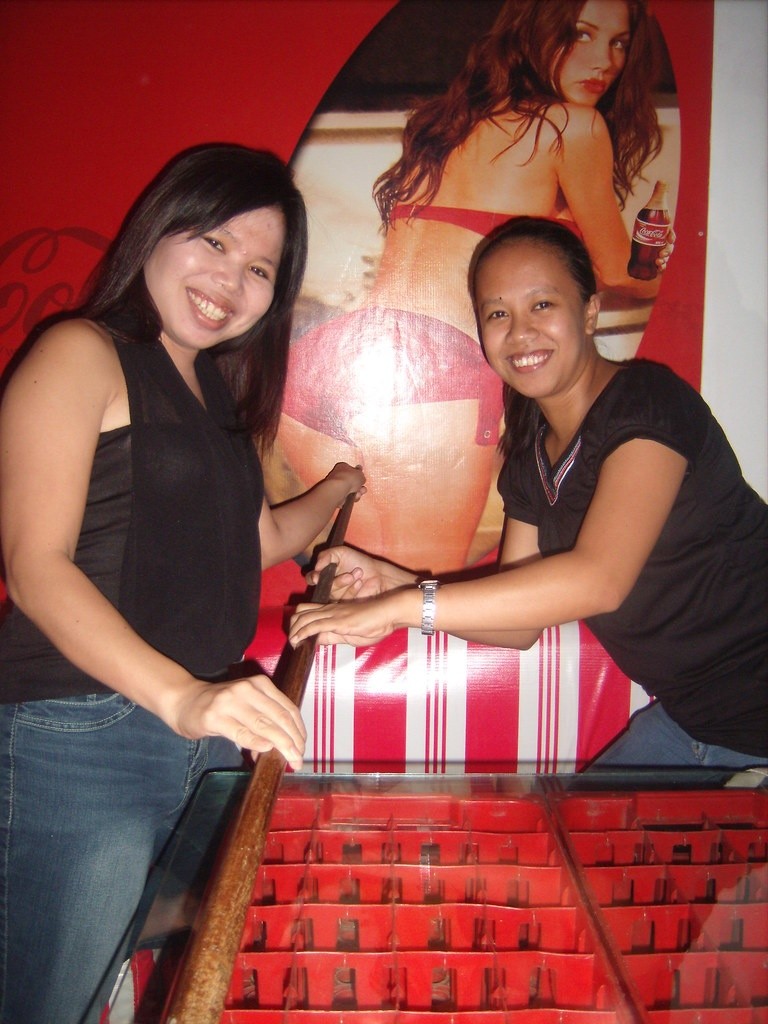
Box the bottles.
[628,181,669,281]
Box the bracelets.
[415,579,442,638]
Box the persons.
[0,139,368,1024]
[286,214,767,773]
[261,1,675,581]
